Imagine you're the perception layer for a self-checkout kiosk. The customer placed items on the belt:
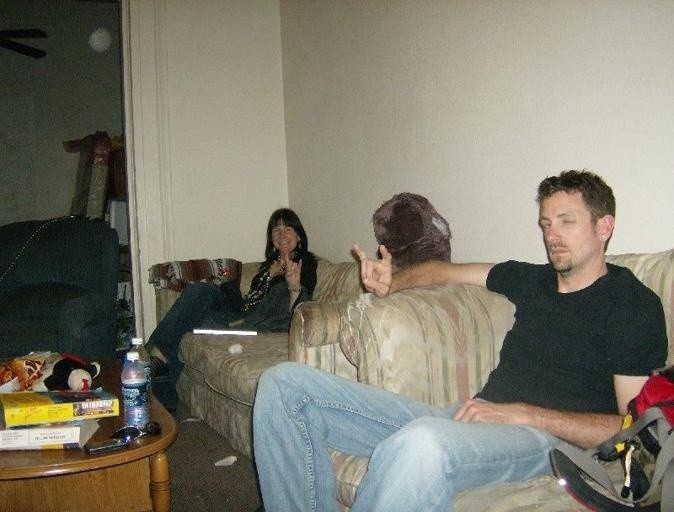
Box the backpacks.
[546,364,673,512]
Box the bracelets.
[287,287,301,293]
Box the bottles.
[120,337,152,430]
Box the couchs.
[327,251,673,512]
[149,250,375,469]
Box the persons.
[140,208,318,418]
[250,168,667,510]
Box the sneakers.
[149,356,170,383]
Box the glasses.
[111,421,162,441]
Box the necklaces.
[243,259,285,311]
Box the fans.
[1,24,47,60]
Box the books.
[0,387,119,428]
[0,417,100,450]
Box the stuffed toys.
[31,357,100,392]
[372,192,451,263]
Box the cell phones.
[85,438,128,455]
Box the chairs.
[0,214,120,361]
